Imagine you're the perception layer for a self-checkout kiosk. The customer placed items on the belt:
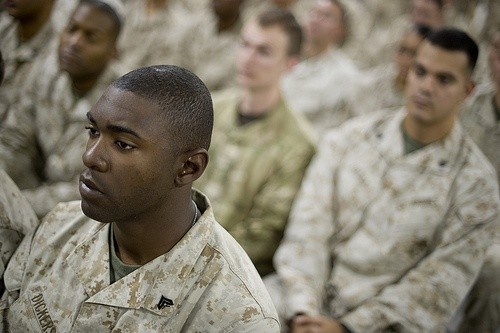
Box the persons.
[0,0,500,333]
[0,64,281,333]
